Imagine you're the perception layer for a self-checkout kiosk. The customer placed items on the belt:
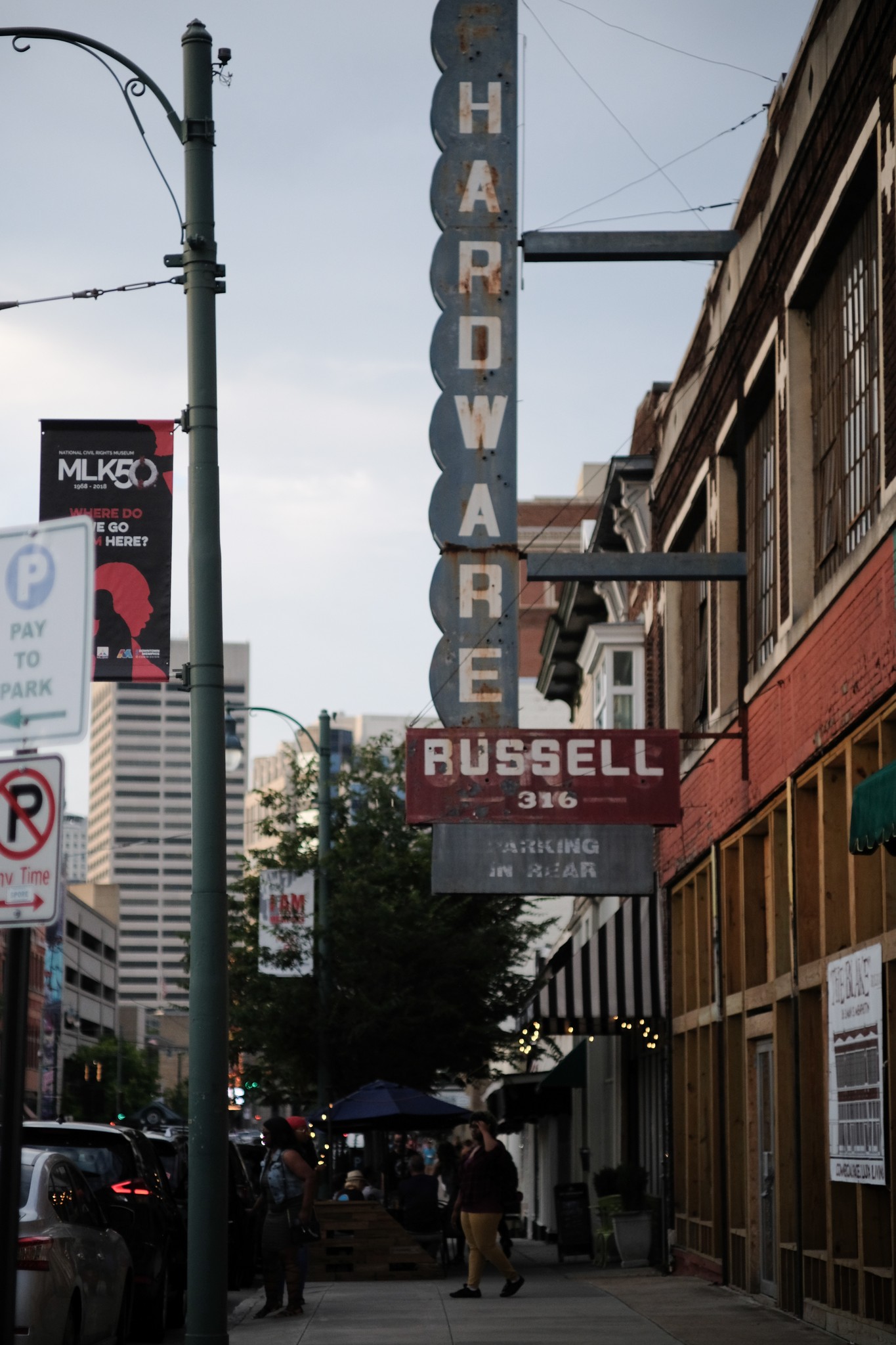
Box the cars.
[18,1146,135,1345]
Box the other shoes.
[253,1302,280,1319]
[450,1283,481,1298]
[499,1276,524,1298]
[276,1305,305,1317]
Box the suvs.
[18,1117,272,1339]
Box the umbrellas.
[307,1077,475,1187]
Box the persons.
[255,1112,526,1317]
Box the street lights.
[220,706,333,1110]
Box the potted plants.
[592,1159,662,1268]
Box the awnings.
[849,760,896,856]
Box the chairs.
[593,1194,624,1269]
